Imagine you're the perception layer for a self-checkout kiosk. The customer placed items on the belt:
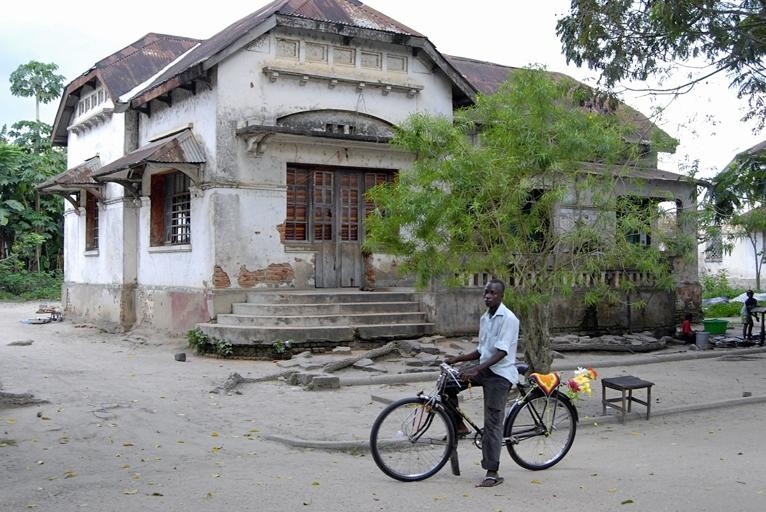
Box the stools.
[601,375,656,426]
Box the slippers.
[442,431,471,441]
[482,477,503,486]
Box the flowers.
[527,367,599,406]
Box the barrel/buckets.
[695,332,711,347]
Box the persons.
[682,313,697,337]
[741,290,760,339]
[438,279,519,487]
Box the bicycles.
[369,362,580,482]
